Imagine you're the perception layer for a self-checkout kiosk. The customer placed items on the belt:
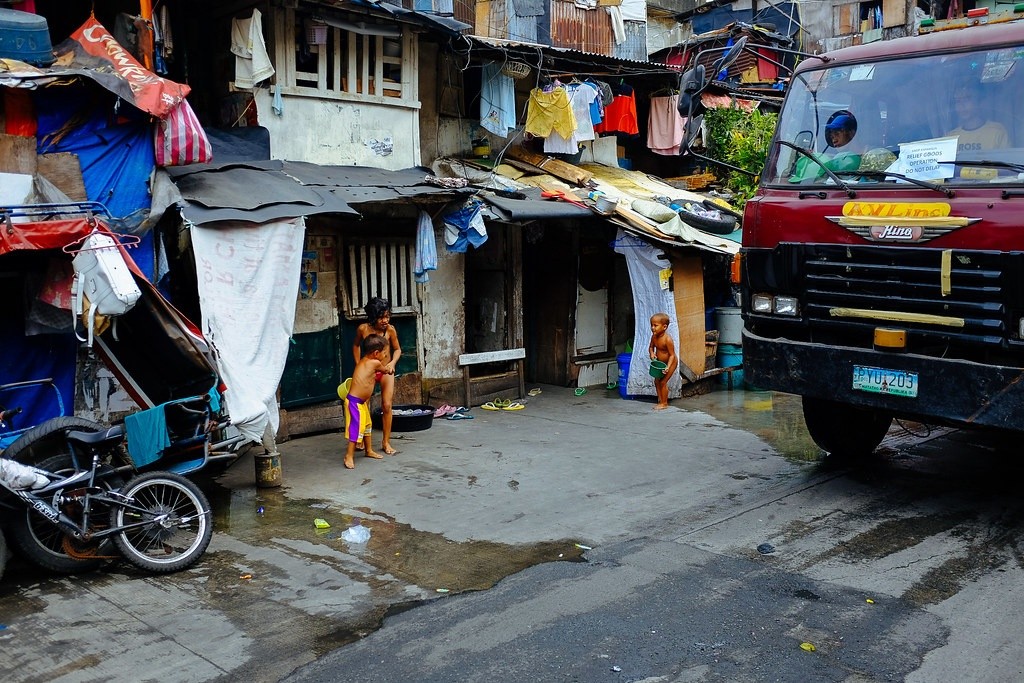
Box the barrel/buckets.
[616,351,643,398]
[714,306,744,344]
[717,344,747,390]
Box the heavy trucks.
[678,13,1024,484]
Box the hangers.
[62,216,142,258]
[484,56,691,101]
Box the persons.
[649,313,678,410]
[343,334,388,469]
[352,296,402,454]
[820,112,880,161]
[937,76,1012,153]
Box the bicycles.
[0,396,216,578]
